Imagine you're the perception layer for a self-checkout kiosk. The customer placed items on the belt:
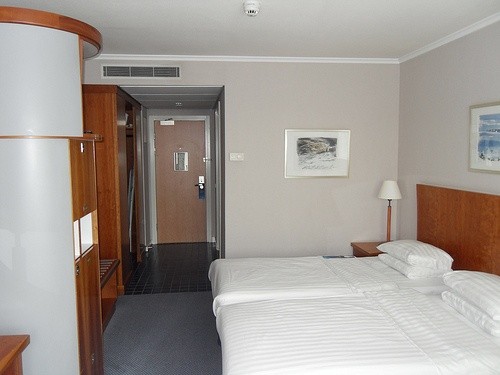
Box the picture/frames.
[468,102,500,174]
[283,128,352,179]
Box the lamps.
[378,180,402,244]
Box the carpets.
[102,291,222,375]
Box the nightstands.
[350,241,385,257]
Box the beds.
[209,183,500,375]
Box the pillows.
[378,253,451,279]
[376,239,452,267]
[442,291,500,339]
[442,270,500,321]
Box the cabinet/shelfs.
[0,6,103,375]
[83,84,148,295]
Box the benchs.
[99,258,120,288]
